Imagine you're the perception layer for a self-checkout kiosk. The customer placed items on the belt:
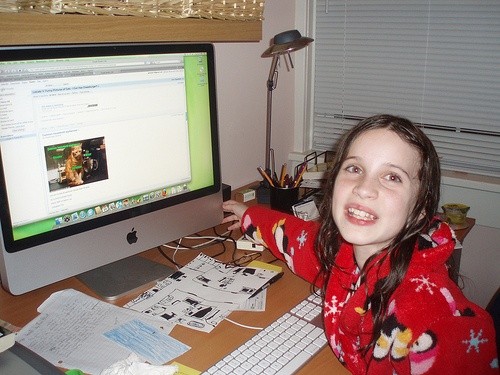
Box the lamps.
[250,29,315,203]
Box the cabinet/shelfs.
[296,151,336,214]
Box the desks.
[0,180,477,375]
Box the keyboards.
[199,288,328,375]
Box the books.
[0,326,15,355]
[300,162,333,188]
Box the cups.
[271,186,299,216]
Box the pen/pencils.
[256,166,274,186]
[290,162,307,189]
[279,163,287,188]
[248,272,284,300]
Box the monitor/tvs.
[0,42,224,301]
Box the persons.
[220,115,500,375]
